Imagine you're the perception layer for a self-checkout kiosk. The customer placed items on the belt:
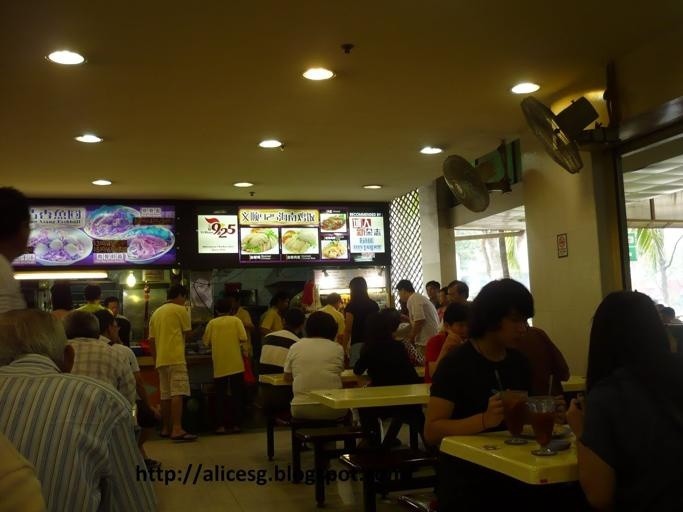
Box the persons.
[49,278,473,446]
[0,186,31,314]
[0,306,161,511]
[513,324,570,398]
[565,290,682,512]
[659,307,681,325]
[424,278,567,512]
[1,432,48,512]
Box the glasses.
[127,271,136,287]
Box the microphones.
[292,406,436,512]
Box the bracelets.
[481,412,487,432]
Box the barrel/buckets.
[161,430,171,438]
[170,433,196,440]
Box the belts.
[502,390,527,445]
[528,396,556,456]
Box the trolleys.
[520,95,603,173]
[442,139,513,212]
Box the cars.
[561,379,587,411]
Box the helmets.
[28,205,176,266]
[240,213,348,260]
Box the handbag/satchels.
[481,412,487,432]
